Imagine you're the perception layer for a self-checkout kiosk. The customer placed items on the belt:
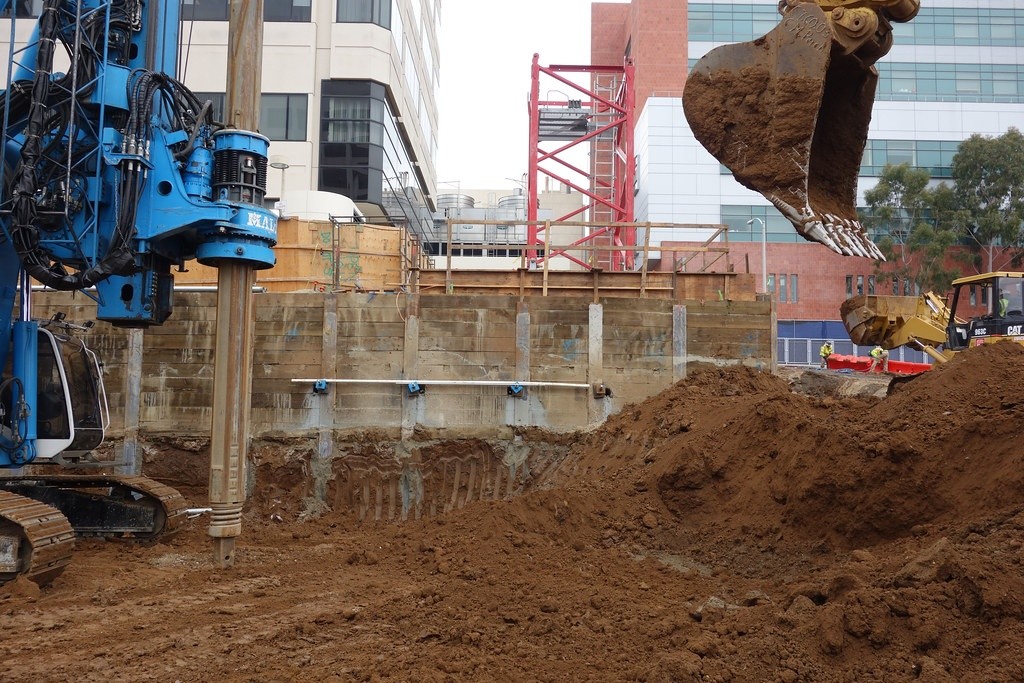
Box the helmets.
[867,350,870,356]
[825,341,831,346]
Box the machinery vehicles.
[0,0,281,589]
[839,272,1024,369]
[683,0,922,262]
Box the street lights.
[747,217,766,293]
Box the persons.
[867,348,889,373]
[819,341,832,369]
[39,366,51,393]
[987,290,1010,319]
